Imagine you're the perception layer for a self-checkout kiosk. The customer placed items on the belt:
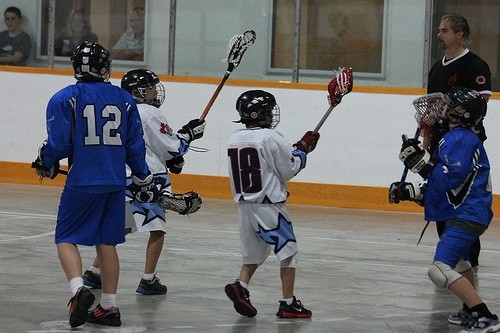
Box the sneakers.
[447,307,472,324]
[82,269,103,289]
[224,280,257,317]
[67,286,96,327]
[136,273,167,295]
[87,304,121,326]
[460,314,500,333]
[276,295,312,318]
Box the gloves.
[388,182,423,204]
[130,171,159,203]
[35,141,60,180]
[399,134,430,173]
[293,130,320,156]
[178,119,206,144]
[165,155,184,175]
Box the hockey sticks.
[198,29,256,122]
[313,68,355,135]
[32,162,203,216]
[399,92,448,183]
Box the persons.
[389,87,500,333]
[83,69,207,294]
[0,7,31,66]
[224,89,320,318]
[423,13,491,266]
[111,7,145,61]
[35,41,159,328]
[54,7,98,56]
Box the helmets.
[71,42,111,84]
[438,85,487,131]
[120,68,166,108]
[237,90,280,128]
